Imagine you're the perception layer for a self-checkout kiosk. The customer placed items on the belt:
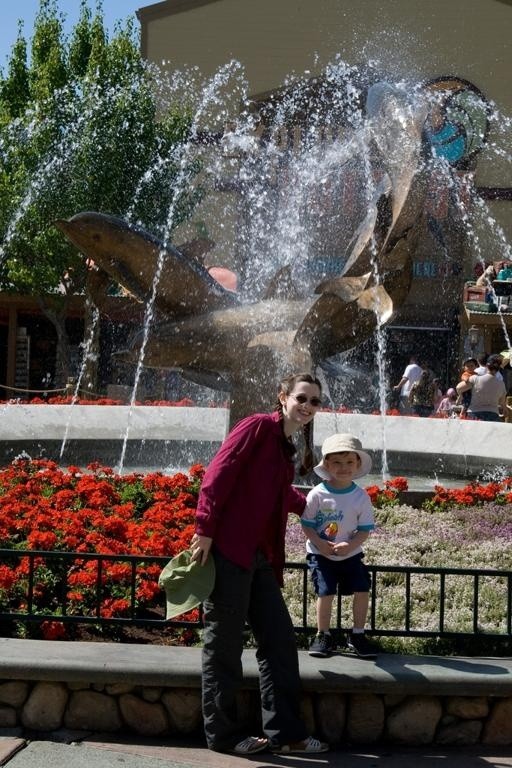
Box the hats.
[313,433,374,483]
[462,356,477,366]
[158,549,216,621]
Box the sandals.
[227,735,268,754]
[269,736,330,753]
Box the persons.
[189,372,330,757]
[298,433,381,659]
[389,347,511,421]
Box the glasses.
[287,391,323,407]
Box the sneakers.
[308,630,333,657]
[346,632,380,658]
